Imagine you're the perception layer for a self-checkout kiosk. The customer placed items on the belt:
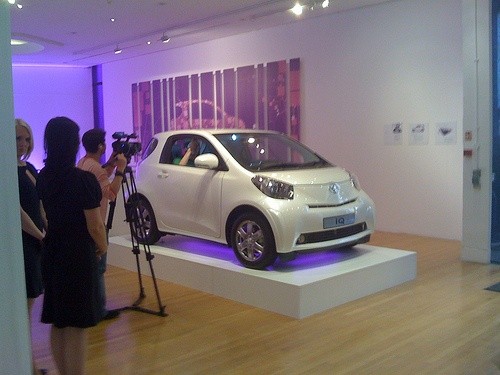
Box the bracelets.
[115,171,124,177]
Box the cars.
[166,100,247,133]
[124,128,375,270]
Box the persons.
[77,128,127,224]
[15,118,48,375]
[172,135,200,167]
[40,117,107,375]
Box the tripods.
[105,158,168,317]
